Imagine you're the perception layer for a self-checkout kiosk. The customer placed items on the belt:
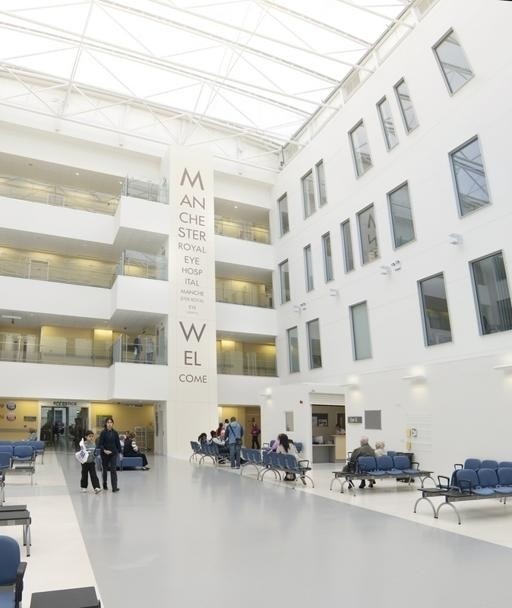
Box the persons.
[197,417,260,470]
[79,430,101,494]
[343,437,374,489]
[100,417,120,492]
[367,441,384,487]
[134,335,156,365]
[120,431,150,471]
[277,433,299,481]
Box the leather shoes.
[373,480,376,484]
[348,482,355,489]
[368,484,373,487]
[359,481,365,488]
[240,457,249,464]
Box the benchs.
[96,453,143,471]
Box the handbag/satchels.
[73,448,90,464]
[235,438,243,446]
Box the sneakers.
[112,488,119,492]
[94,487,102,494]
[80,487,88,493]
[103,487,108,490]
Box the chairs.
[413,459,512,527]
[1,438,49,607]
[330,451,437,496]
[239,446,313,489]
[188,440,239,467]
